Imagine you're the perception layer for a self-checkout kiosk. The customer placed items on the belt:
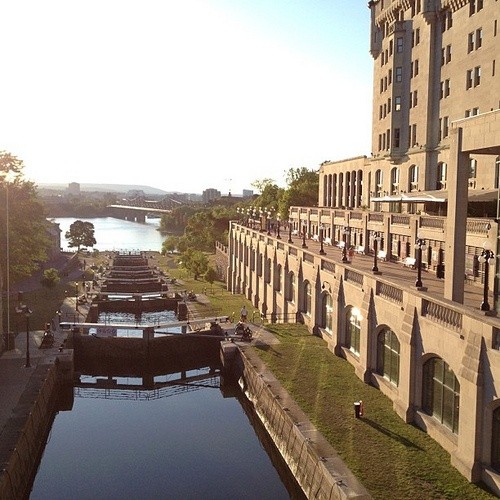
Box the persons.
[342,247,354,261]
[241,305,248,321]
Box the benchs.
[335,241,345,249]
[373,250,387,262]
[19,302,28,312]
[291,230,299,235]
[352,245,365,255]
[15,305,25,318]
[323,237,332,246]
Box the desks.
[309,234,319,243]
[396,256,417,270]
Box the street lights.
[20,304,33,368]
[236,207,382,276]
[477,240,496,311]
[413,238,427,287]
